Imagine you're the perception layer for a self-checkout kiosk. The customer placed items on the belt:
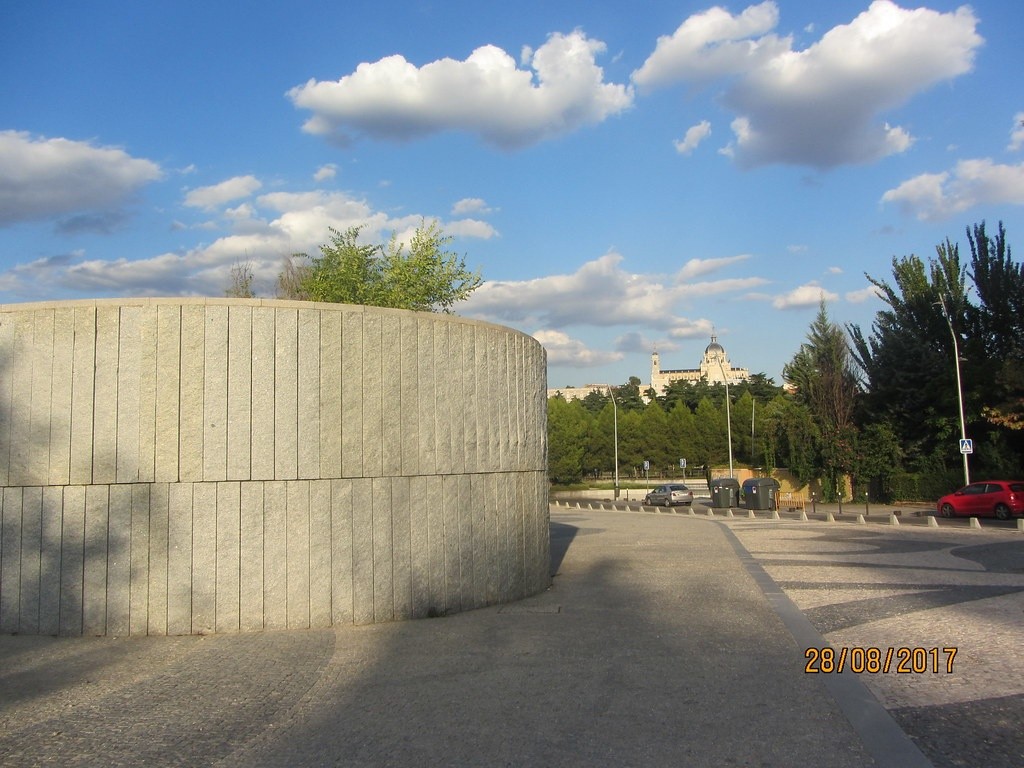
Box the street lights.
[935,291,971,486]
[606,381,618,488]
[715,349,733,478]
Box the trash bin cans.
[741,477,780,511]
[708,478,740,509]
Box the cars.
[935,481,1024,519]
[646,483,694,506]
